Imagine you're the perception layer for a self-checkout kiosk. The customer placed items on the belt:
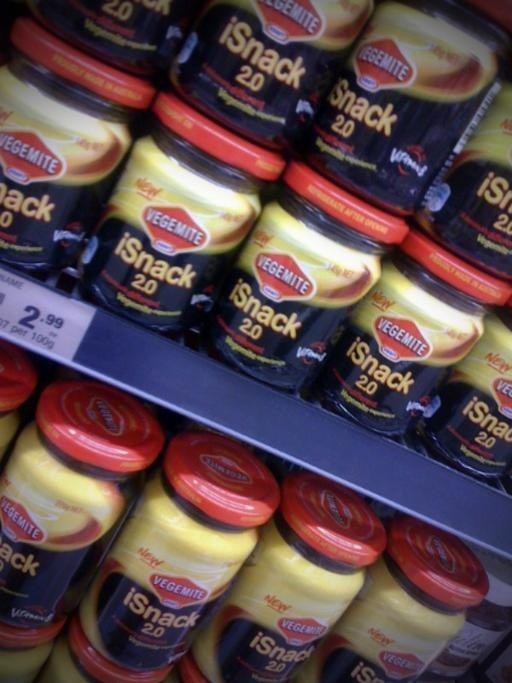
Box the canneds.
[0,1,512,281]
[0,15,512,480]
[1,340,511,682]
